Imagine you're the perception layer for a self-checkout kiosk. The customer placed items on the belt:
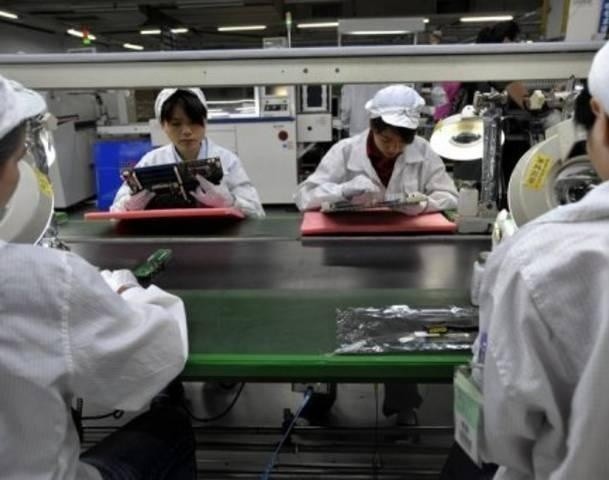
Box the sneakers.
[292,383,337,426]
[394,407,419,426]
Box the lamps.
[429,106,508,235]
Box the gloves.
[340,174,381,197]
[189,174,234,207]
[113,188,156,211]
[99,268,139,295]
[392,201,427,216]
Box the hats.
[587,40,609,117]
[154,86,207,122]
[363,84,426,129]
[0,75,48,141]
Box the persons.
[290,83,459,428]
[104,86,267,224]
[0,73,191,480]
[466,37,609,479]
[430,19,533,209]
